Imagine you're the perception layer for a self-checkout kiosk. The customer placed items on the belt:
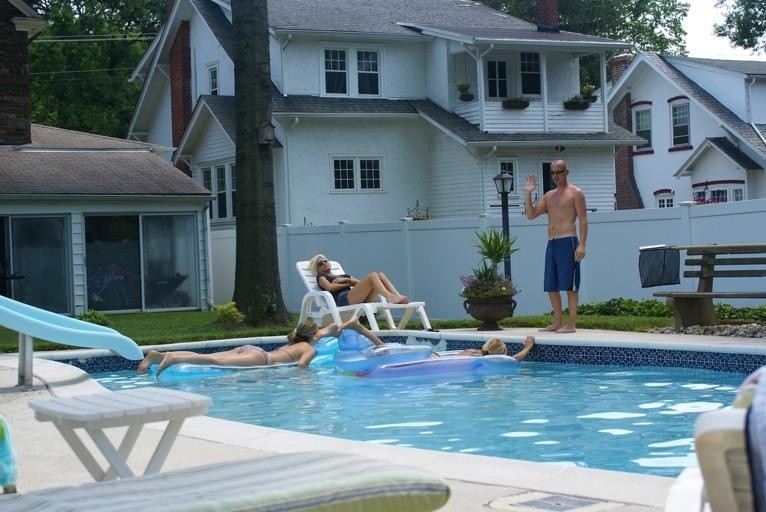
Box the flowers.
[457,262,522,297]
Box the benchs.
[652,243,766,333]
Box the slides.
[0,296,144,360]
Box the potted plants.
[503,96,529,109]
[583,85,597,103]
[454,80,475,101]
[564,96,589,109]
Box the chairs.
[296,261,433,333]
[0,449,452,512]
[691,365,766,512]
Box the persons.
[137,316,319,380]
[308,253,410,306]
[313,314,536,361]
[522,159,590,335]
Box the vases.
[464,297,516,332]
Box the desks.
[28,386,211,481]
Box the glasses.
[551,168,565,175]
[317,259,327,268]
